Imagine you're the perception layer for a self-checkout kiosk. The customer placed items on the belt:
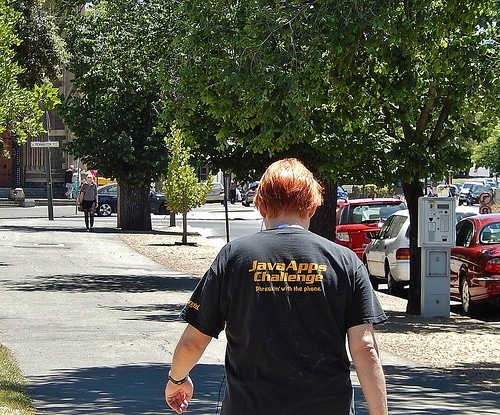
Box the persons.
[165,158,388,415]
[77,174,98,231]
[65,164,74,198]
[230,179,236,204]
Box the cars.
[241,183,258,206]
[337,186,348,199]
[197,182,224,205]
[396,178,500,205]
[450,214,500,316]
[78,183,170,216]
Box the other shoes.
[86,228,89,232]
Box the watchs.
[168,370,187,384]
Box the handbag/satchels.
[79,192,85,203]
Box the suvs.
[335,197,408,259]
[362,209,411,294]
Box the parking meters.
[417,197,456,318]
